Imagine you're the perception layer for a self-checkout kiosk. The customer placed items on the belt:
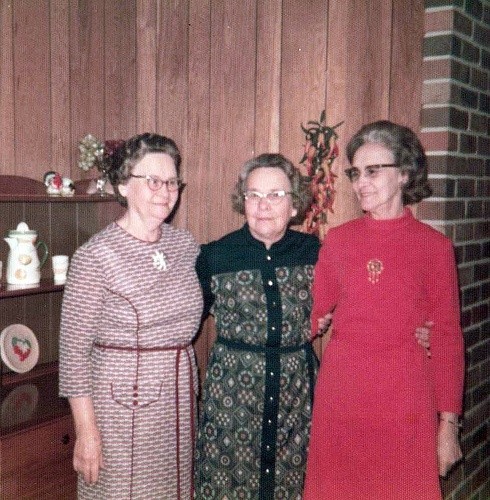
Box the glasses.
[129,173,182,193]
[343,163,400,183]
[244,190,293,205]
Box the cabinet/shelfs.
[0,174,128,500]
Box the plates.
[0,324,40,374]
[0,384,39,431]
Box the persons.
[195,152,434,500]
[302,119,465,500]
[58,132,334,500]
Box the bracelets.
[439,415,463,429]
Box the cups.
[52,255,69,286]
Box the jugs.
[2,221,49,285]
